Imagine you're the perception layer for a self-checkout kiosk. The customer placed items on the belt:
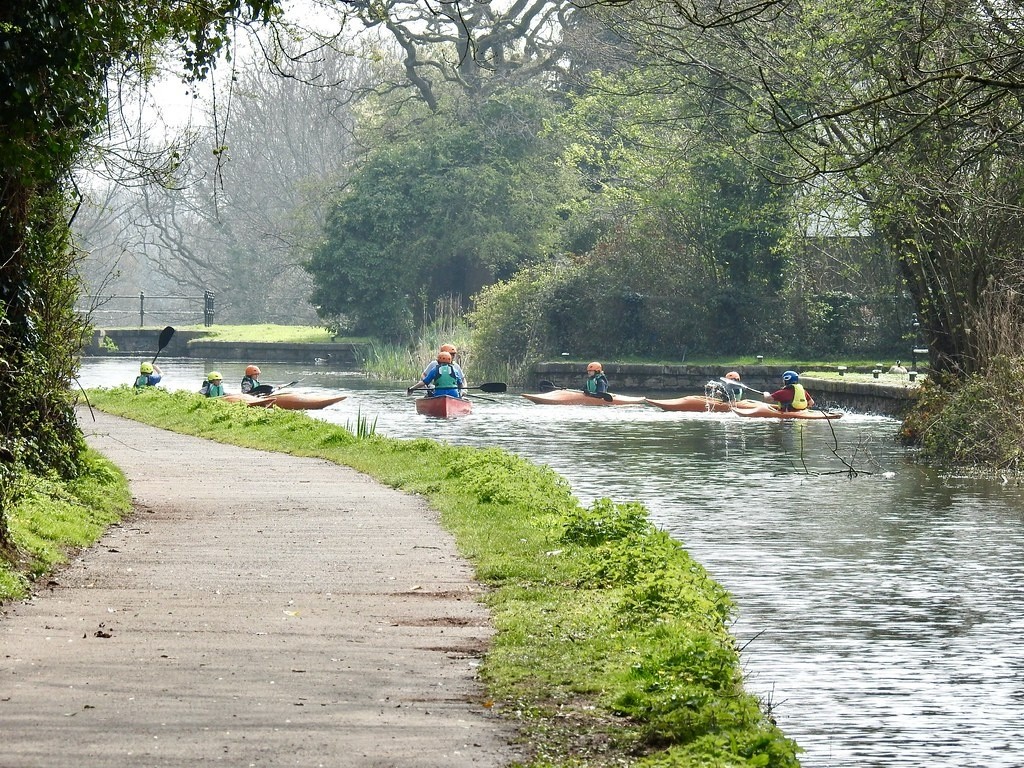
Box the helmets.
[782,371,799,385]
[587,361,601,373]
[208,371,222,381]
[437,345,455,364]
[140,362,153,375]
[245,364,260,377]
[726,370,741,381]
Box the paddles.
[705,378,732,403]
[407,381,508,394]
[149,325,176,365]
[719,375,833,412]
[460,390,504,405]
[537,379,614,403]
[255,380,299,397]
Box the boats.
[522,388,647,405]
[730,403,844,420]
[415,395,473,418]
[216,392,277,408]
[646,393,778,412]
[266,393,348,410]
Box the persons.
[196,370,233,397]
[241,365,262,395]
[579,361,608,399]
[763,370,815,413]
[407,351,467,399]
[707,372,743,403]
[421,344,469,397]
[133,361,164,387]
